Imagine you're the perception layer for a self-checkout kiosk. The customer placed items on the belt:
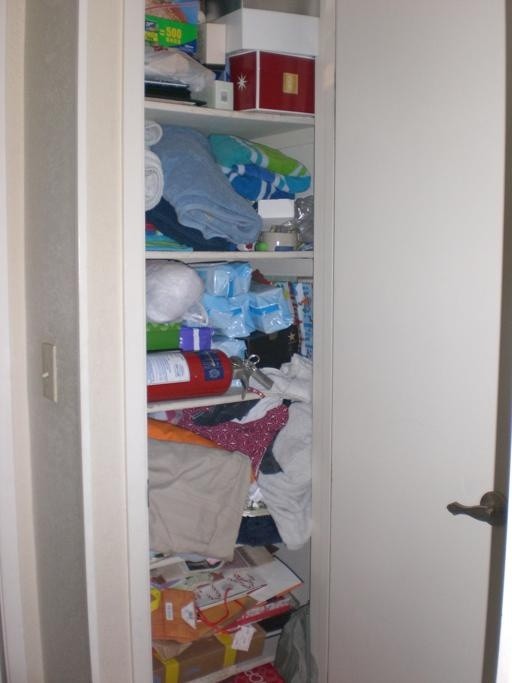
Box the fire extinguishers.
[146,349,260,400]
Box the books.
[144,79,208,107]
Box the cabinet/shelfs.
[76,0,512,683]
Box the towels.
[144,119,312,251]
[230,352,313,551]
